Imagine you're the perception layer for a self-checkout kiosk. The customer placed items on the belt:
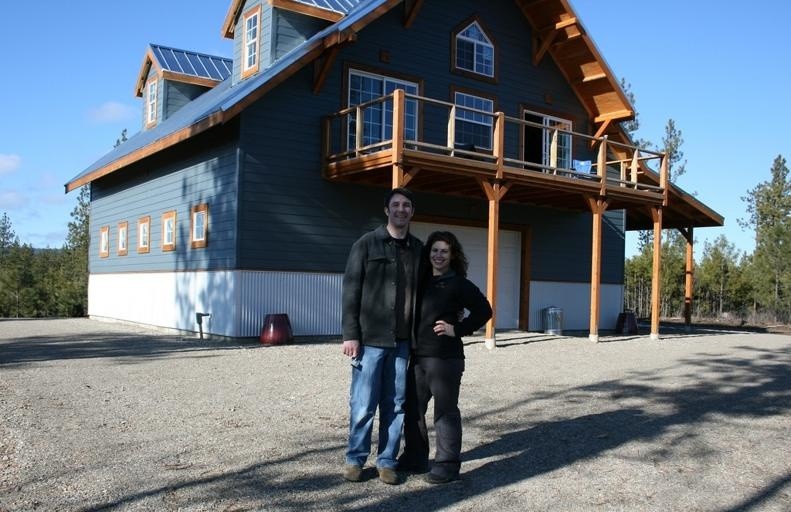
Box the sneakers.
[376,463,400,485]
[343,462,362,482]
[397,451,428,474]
[425,468,462,484]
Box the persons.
[398,229,496,484]
[338,187,427,489]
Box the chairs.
[569,158,602,182]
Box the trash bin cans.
[542,306,564,336]
